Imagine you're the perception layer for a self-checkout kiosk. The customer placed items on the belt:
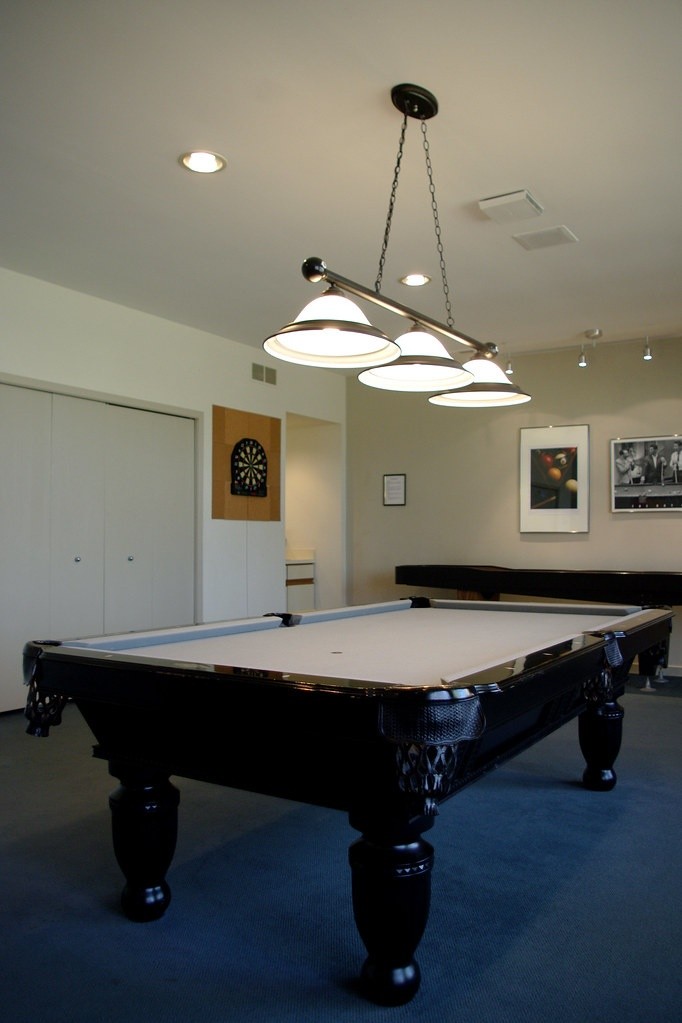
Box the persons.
[670,441,682,508]
[616,447,642,508]
[641,444,667,508]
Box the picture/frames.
[610,435,682,513]
[519,424,589,534]
[383,473,406,506]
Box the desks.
[23,597,674,1007]
[394,565,682,692]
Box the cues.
[674,463,678,484]
[630,465,633,485]
[661,462,665,485]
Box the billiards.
[534,449,542,458]
[615,487,681,494]
[546,467,561,483]
[554,453,570,469]
[539,454,554,470]
[565,479,577,493]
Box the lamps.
[578,345,587,367]
[261,82,531,409]
[505,361,513,375]
[644,337,652,361]
